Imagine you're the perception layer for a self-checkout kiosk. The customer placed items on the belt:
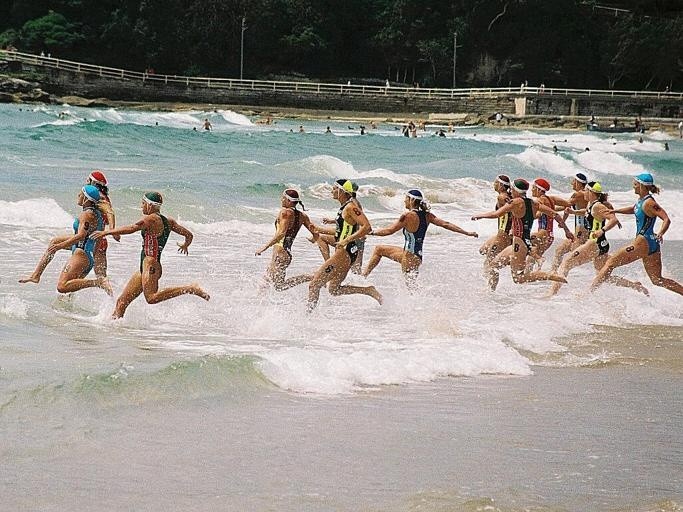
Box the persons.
[349,119,455,138]
[255,190,319,292]
[345,78,352,95]
[85,172,121,288]
[287,123,332,135]
[59,112,69,119]
[18,186,113,296]
[471,172,683,298]
[89,192,210,319]
[307,179,383,309]
[148,65,154,78]
[363,190,478,292]
[478,113,683,151]
[264,118,277,126]
[317,183,369,274]
[7,41,19,55]
[386,78,390,89]
[201,119,212,131]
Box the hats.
[284,189,301,202]
[634,173,654,187]
[405,189,423,201]
[512,178,529,193]
[585,181,602,194]
[142,191,163,207]
[89,171,108,186]
[533,178,550,192]
[81,184,101,203]
[351,181,359,193]
[496,174,510,186]
[334,178,353,196]
[575,173,587,184]
